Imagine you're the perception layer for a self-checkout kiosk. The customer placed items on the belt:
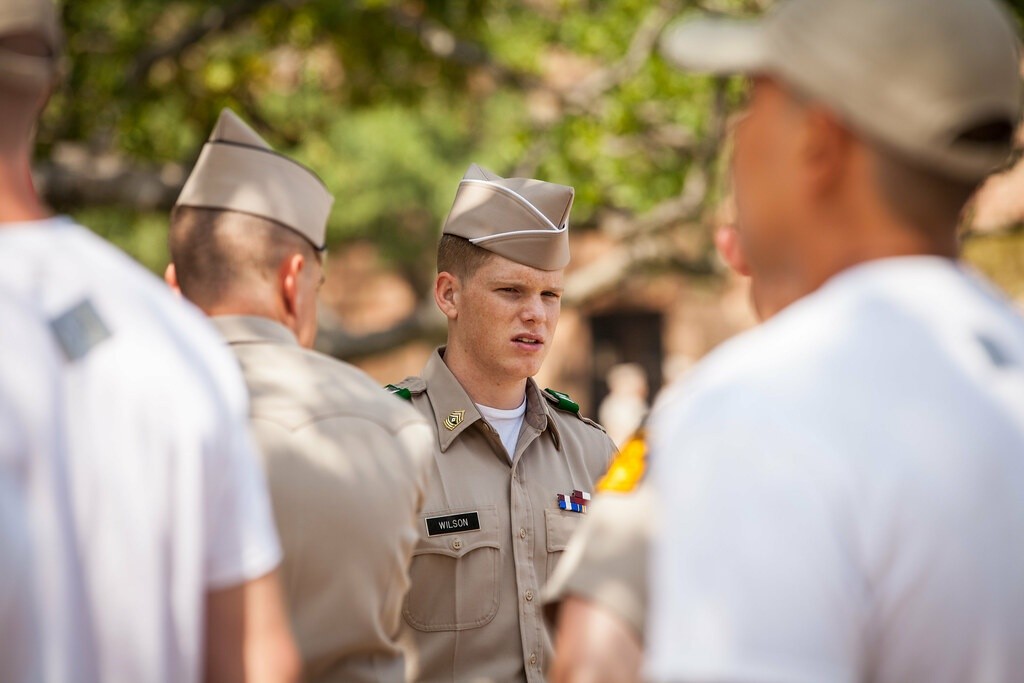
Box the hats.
[176,107,335,264]
[658,0,1024,179]
[443,159,575,271]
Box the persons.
[535,0,1023,683]
[0,0,300,682]
[165,110,434,682]
[383,158,622,683]
[598,358,649,453]
